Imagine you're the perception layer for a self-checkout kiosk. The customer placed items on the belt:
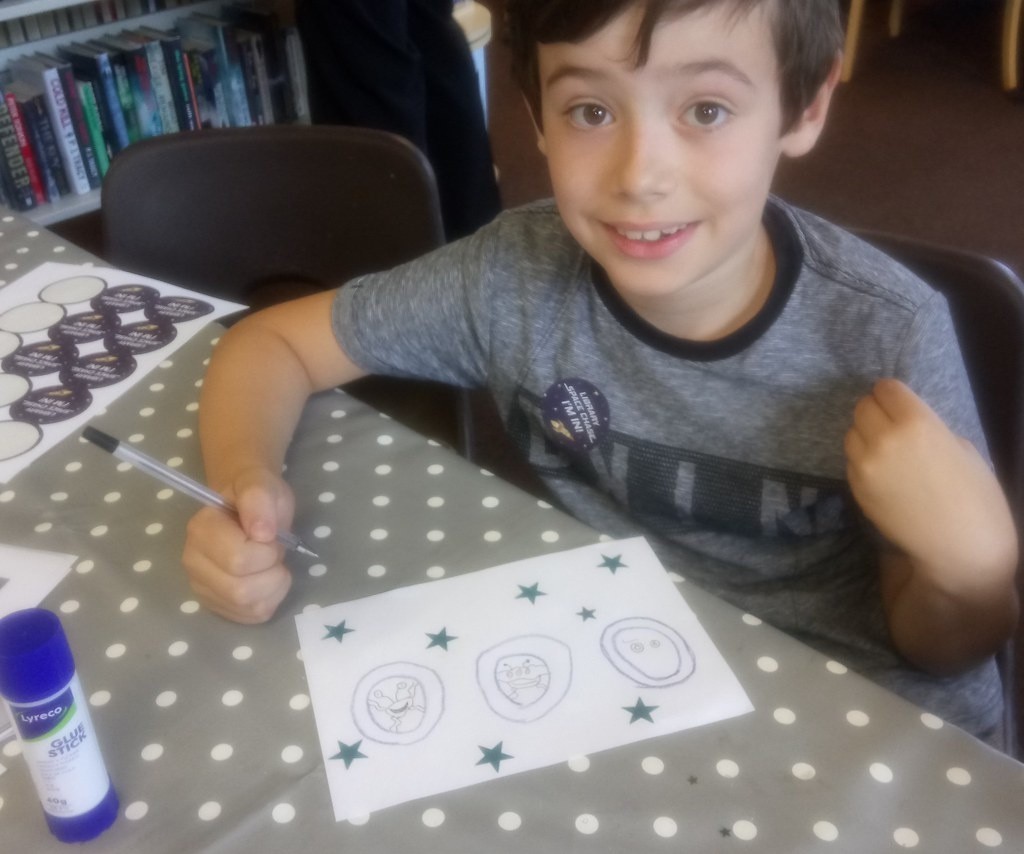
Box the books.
[0,11,299,213]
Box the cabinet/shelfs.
[0,0,313,226]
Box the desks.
[0,210,1024,854]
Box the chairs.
[101,126,448,452]
[840,228,1024,551]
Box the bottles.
[0,608,119,841]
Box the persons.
[296,0,501,244]
[181,0,1019,755]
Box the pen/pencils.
[85,424,319,561]
